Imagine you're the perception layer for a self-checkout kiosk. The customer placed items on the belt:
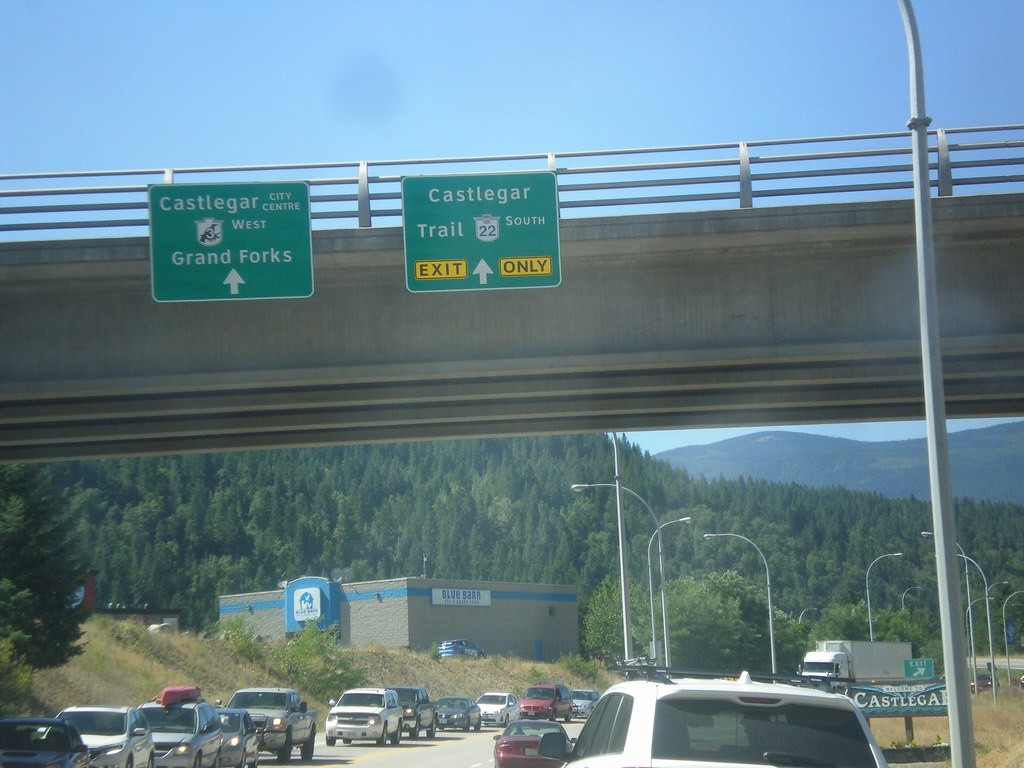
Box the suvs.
[136,698,222,768]
[0,716,93,768]
[563,666,899,768]
[437,639,485,659]
[519,682,573,721]
[326,687,404,745]
[38,705,159,768]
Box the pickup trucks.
[388,686,440,739]
[216,686,318,764]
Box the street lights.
[922,530,1024,704]
[702,532,779,683]
[571,482,693,667]
[866,552,921,641]
[797,607,818,640]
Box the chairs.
[8,730,31,748]
[653,715,695,759]
[45,732,65,748]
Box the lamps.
[246,604,250,609]
[374,591,379,598]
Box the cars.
[970,673,999,695]
[1020,673,1024,691]
[475,692,522,728]
[571,690,600,718]
[434,697,482,731]
[213,706,262,768]
[492,720,577,768]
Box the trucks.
[796,639,914,690]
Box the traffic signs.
[150,181,314,300]
[401,172,562,292]
